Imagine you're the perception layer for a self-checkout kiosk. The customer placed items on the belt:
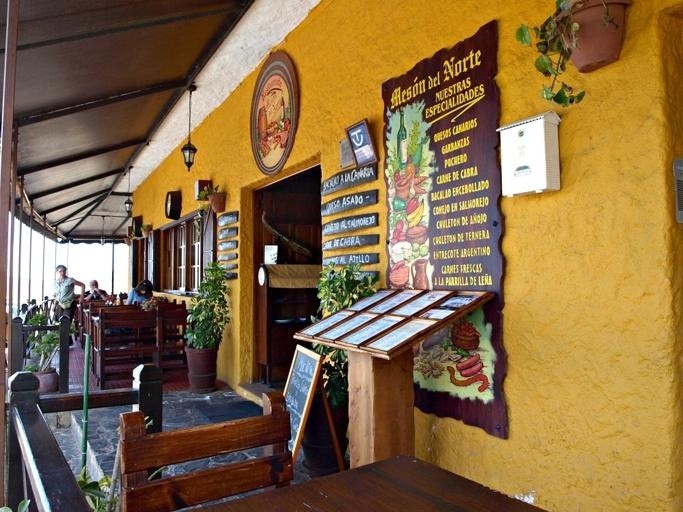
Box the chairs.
[110,393,296,510]
[76,293,187,389]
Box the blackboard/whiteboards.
[282,343,323,466]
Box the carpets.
[194,400,263,423]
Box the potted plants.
[300,259,380,470]
[23,313,78,394]
[512,0,634,109]
[199,184,226,214]
[140,224,153,239]
[181,261,232,394]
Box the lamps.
[179,82,199,173]
[125,165,134,216]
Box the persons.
[126,279,154,305]
[83,280,108,301]
[55,264,85,351]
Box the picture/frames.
[343,118,379,169]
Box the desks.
[184,452,549,512]
